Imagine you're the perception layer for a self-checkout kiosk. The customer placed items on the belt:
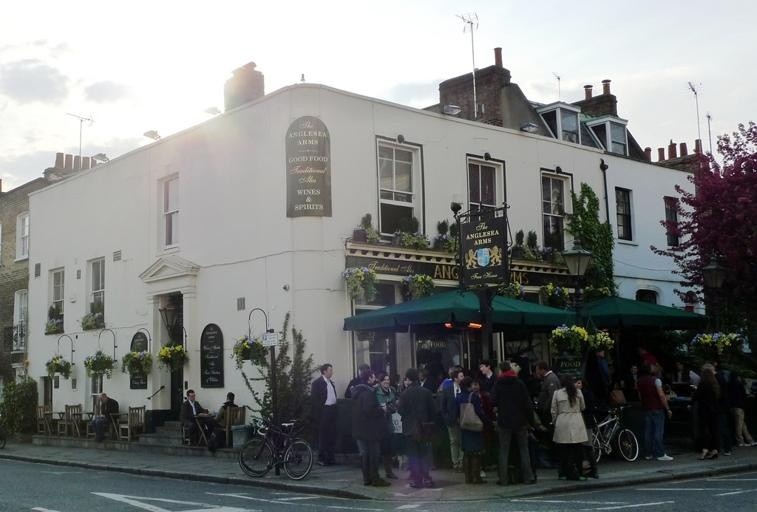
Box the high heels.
[696,448,718,461]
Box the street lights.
[703,254,727,336]
[562,239,592,391]
[236,308,281,475]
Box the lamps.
[144,129,162,143]
[91,153,110,164]
[203,106,222,116]
[42,167,67,181]
[439,101,462,117]
[299,73,307,83]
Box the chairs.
[32,396,246,446]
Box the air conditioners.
[519,122,539,135]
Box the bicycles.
[0,414,8,449]
[238,416,313,481]
[583,406,642,469]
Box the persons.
[92,393,118,441]
[688,358,755,460]
[439,358,598,486]
[310,363,338,466]
[182,389,210,445]
[207,391,238,440]
[346,364,440,488]
[585,349,689,460]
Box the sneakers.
[318,456,599,488]
[724,442,757,457]
[646,452,675,462]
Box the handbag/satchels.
[416,422,439,443]
[390,411,403,434]
[458,403,484,432]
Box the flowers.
[47,354,72,380]
[158,341,190,373]
[692,328,741,356]
[400,272,436,299]
[499,279,612,299]
[549,321,615,353]
[121,351,154,374]
[83,351,116,379]
[343,266,380,304]
[232,334,270,368]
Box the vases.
[353,283,367,304]
[411,287,423,300]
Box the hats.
[499,361,511,371]
